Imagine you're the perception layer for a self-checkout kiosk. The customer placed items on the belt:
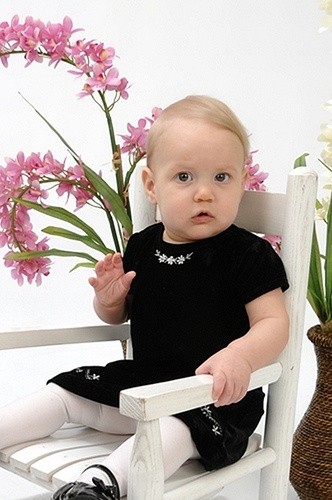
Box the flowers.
[0,15,282,316]
[293,1,332,335]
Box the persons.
[0,97,288,500]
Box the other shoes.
[53,464,120,500]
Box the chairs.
[0,167,319,500]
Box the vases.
[289,323,332,500]
